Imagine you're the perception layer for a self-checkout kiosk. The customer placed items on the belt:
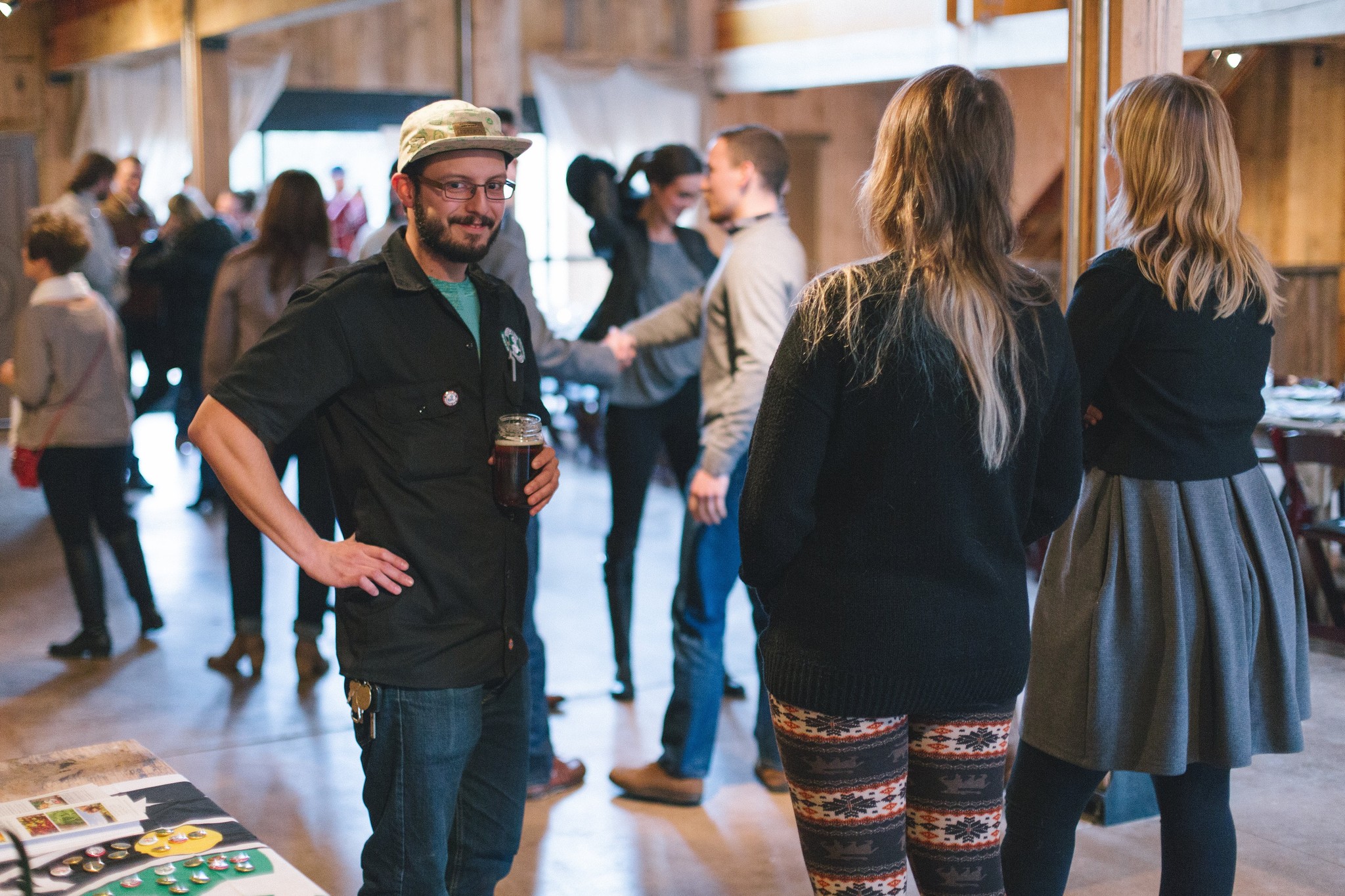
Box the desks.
[0,735,324,895]
[1253,387,1345,607]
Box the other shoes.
[610,684,636,703]
[612,762,704,806]
[526,755,585,800]
[185,489,215,515]
[129,475,154,490]
[140,606,164,632]
[723,672,745,699]
[756,763,791,793]
[50,622,112,658]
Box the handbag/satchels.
[11,444,45,489]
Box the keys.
[346,680,381,739]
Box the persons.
[610,125,807,802]
[0,207,166,659]
[202,170,349,680]
[741,65,1086,896]
[57,152,257,513]
[480,109,635,801]
[324,167,368,255]
[188,99,562,896]
[578,144,746,698]
[1000,72,1312,896]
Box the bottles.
[491,412,545,509]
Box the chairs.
[1257,420,1345,642]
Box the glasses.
[415,173,516,200]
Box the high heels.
[208,632,265,673]
[296,634,330,681]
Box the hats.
[397,99,533,176]
